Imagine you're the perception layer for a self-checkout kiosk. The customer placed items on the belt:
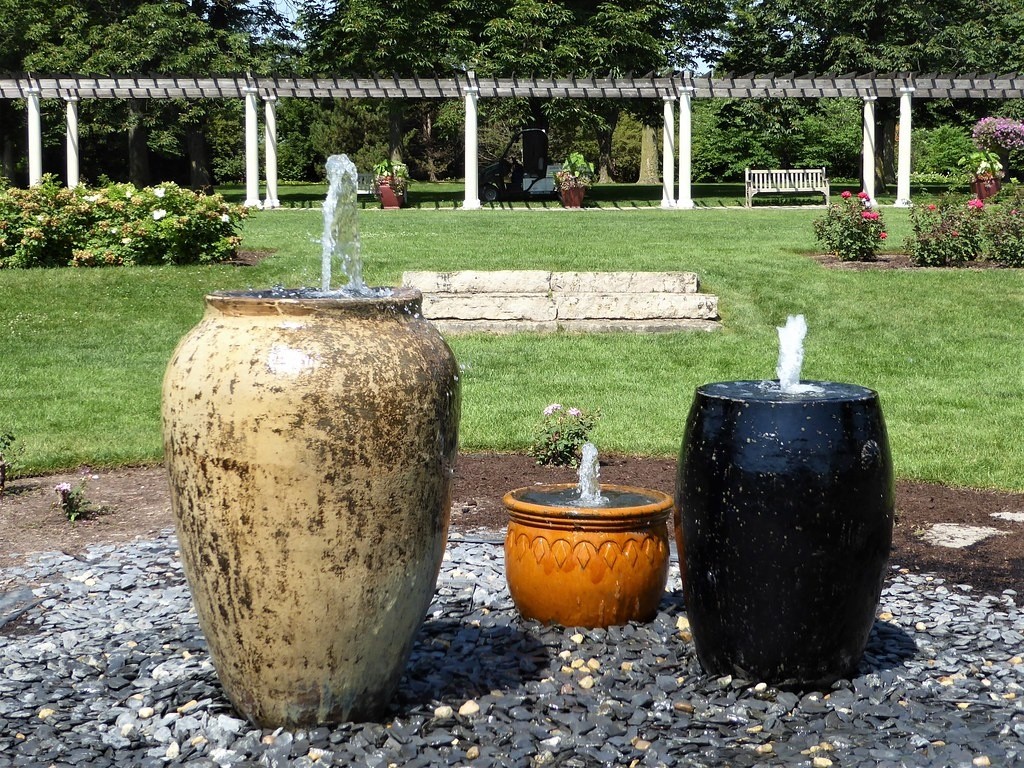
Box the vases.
[972,178,1001,199]
[379,181,404,209]
[559,187,585,208]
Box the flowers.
[377,159,410,196]
[54,479,92,526]
[958,152,1005,184]
[553,152,595,189]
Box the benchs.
[744,167,830,208]
